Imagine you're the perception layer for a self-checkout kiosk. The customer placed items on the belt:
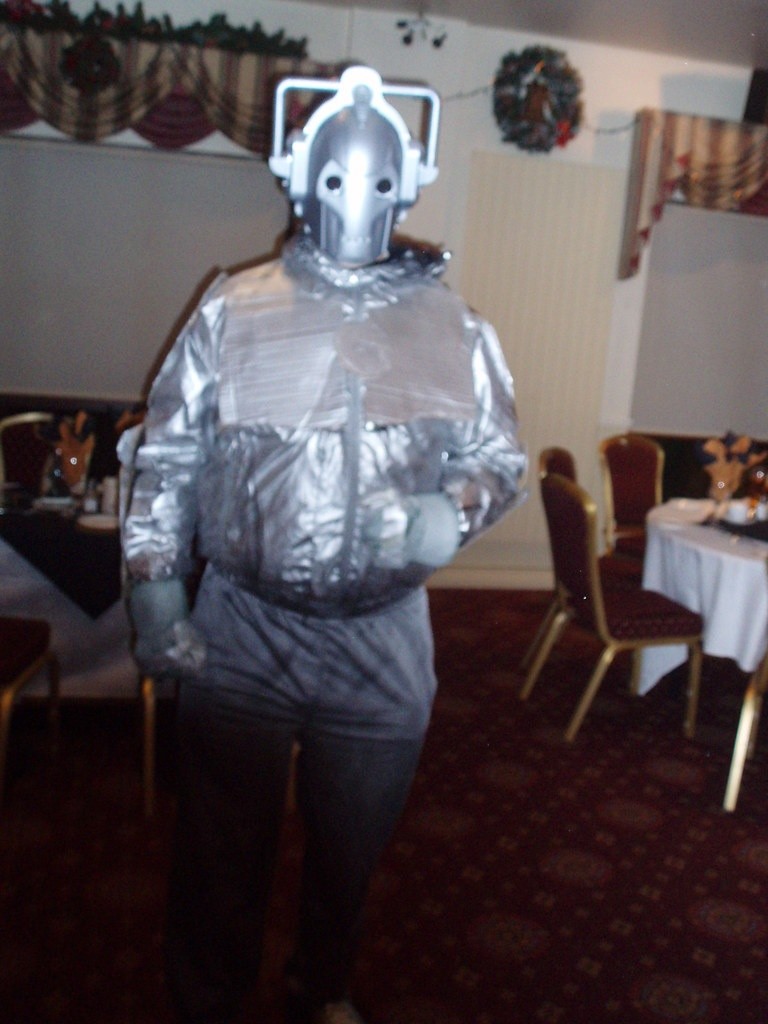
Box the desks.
[0,505,177,696]
[642,496,768,814]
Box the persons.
[122,68,532,1023]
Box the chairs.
[519,433,706,743]
[114,409,305,818]
[0,616,60,782]
[1,415,54,491]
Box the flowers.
[493,44,583,153]
[0,0,310,59]
